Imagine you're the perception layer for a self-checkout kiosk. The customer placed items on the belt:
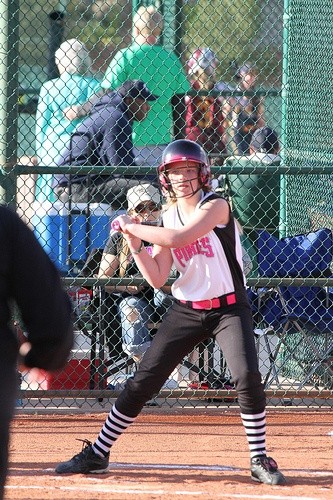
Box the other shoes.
[163,375,236,392]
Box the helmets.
[158,139,211,173]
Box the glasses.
[134,203,163,214]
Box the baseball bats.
[110,219,161,231]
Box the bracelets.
[130,241,144,255]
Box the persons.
[184,47,313,242]
[56,139,286,485]
[62,7,191,167]
[51,80,161,209]
[0,202,73,500]
[34,36,101,200]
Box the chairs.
[90,228,333,406]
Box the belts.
[180,293,236,311]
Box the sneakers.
[55,441,111,475]
[249,458,287,486]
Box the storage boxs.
[32,202,113,272]
[28,349,106,398]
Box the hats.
[117,79,160,101]
[125,185,162,212]
[250,127,279,149]
[186,46,221,74]
